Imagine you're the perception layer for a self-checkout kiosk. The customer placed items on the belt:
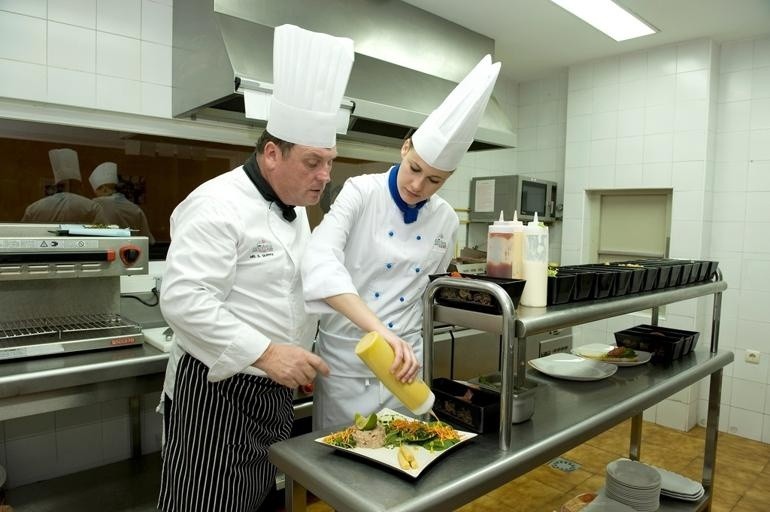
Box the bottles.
[356,330,439,422]
[485,208,549,309]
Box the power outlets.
[745,349,759,363]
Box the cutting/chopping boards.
[142,325,176,354]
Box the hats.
[266,22,354,149]
[89,162,119,190]
[48,148,83,183]
[411,54,502,172]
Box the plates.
[577,495,635,510]
[618,456,706,501]
[308,406,481,482]
[526,351,618,385]
[571,340,657,366]
[605,460,663,511]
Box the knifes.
[162,325,173,342]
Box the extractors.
[171,0,517,153]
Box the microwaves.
[468,176,558,224]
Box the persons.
[301,133,466,444]
[17,149,93,224]
[86,161,151,243]
[155,131,338,512]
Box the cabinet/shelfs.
[267,266,735,512]
